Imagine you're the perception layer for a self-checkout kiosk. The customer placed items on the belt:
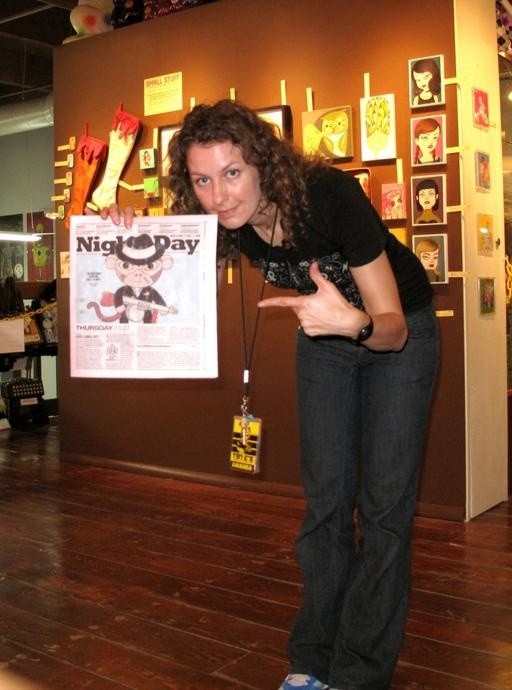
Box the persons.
[99,100,440,688]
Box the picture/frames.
[478,275,498,318]
[408,114,448,169]
[409,175,447,228]
[408,54,447,111]
[247,105,293,138]
[474,151,491,193]
[156,122,185,186]
[472,88,491,132]
[410,233,450,282]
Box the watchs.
[352,315,375,347]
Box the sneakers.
[276,671,329,690]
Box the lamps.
[0,133,41,243]
